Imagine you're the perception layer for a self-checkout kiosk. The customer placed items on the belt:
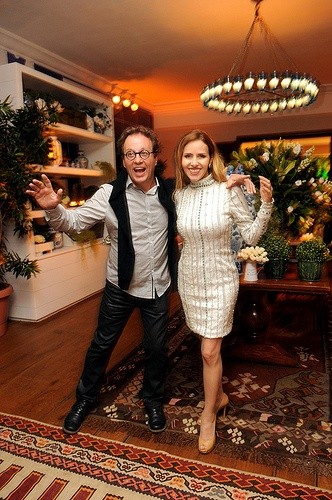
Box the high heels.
[197,391,229,425]
[198,412,216,454]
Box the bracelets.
[260,197,274,205]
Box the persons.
[172,129,274,454]
[26,125,256,434]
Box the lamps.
[199,1,320,117]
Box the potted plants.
[261,236,292,280]
[296,238,330,282]
[0,91,63,337]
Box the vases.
[245,261,257,281]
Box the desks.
[238,260,332,367]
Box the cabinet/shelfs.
[0,62,116,324]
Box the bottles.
[49,227,63,249]
[55,179,68,196]
[68,178,80,198]
[73,104,83,127]
[48,136,63,168]
[65,159,80,168]
[75,151,88,169]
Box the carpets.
[0,412,332,500]
[60,299,332,476]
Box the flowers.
[238,245,270,265]
[230,138,331,240]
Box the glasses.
[123,149,157,161]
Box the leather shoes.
[63,399,96,433]
[146,403,167,432]
